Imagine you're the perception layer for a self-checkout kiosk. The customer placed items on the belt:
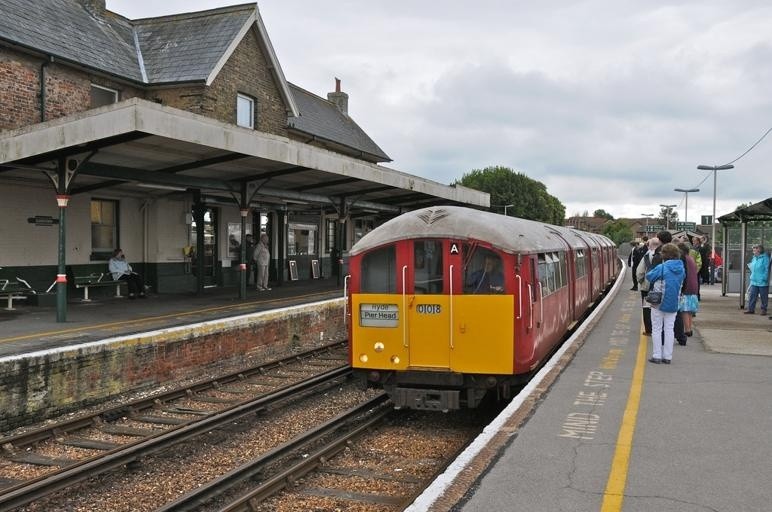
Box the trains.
[343,206,618,415]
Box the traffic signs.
[645,225,663,232]
[676,221,697,232]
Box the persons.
[627,231,723,364]
[108,248,146,299]
[252,234,272,291]
[744,244,770,315]
[466,255,503,293]
[238,234,254,286]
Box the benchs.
[73,280,127,301]
[0,289,25,310]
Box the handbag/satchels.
[645,291,661,304]
[745,286,752,301]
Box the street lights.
[697,163,735,285]
[661,203,676,231]
[642,213,654,236]
[504,204,515,214]
[674,188,701,221]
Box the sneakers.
[630,286,637,290]
[679,331,692,346]
[648,358,671,364]
[642,331,652,336]
[744,311,766,315]
[257,286,272,291]
[129,293,147,300]
